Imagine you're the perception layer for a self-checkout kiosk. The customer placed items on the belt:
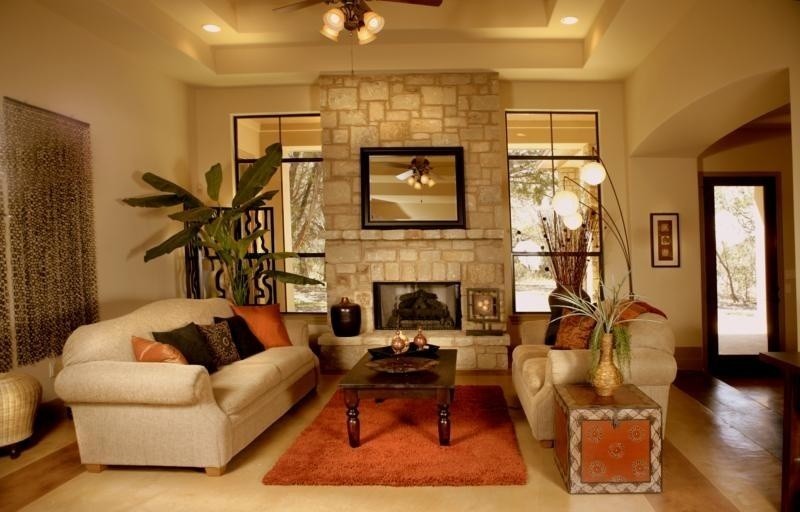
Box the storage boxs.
[553,384,662,495]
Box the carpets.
[263,386,528,488]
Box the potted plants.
[538,204,608,309]
[548,268,663,399]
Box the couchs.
[54,296,319,478]
[512,309,679,448]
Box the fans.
[375,155,456,181]
[274,0,441,17]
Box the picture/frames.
[650,212,681,268]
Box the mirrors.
[360,145,466,230]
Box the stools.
[1,371,44,460]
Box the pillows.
[198,322,239,367]
[553,307,596,352]
[230,304,293,347]
[150,322,214,373]
[133,335,186,364]
[214,315,265,361]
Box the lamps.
[320,2,385,78]
[409,173,436,189]
[552,147,634,300]
[465,286,504,337]
